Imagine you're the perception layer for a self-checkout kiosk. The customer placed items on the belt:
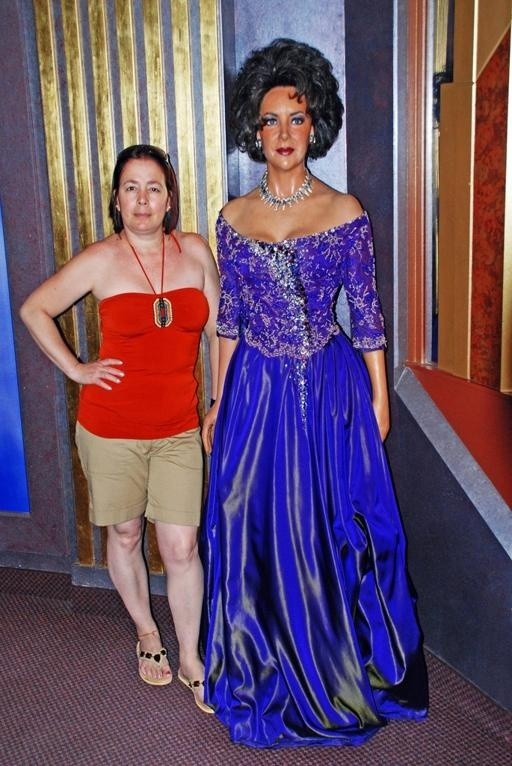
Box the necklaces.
[260,169,315,212]
[123,228,184,331]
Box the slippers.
[178,667,215,714]
[135,641,172,685]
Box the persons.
[200,36,431,753]
[19,143,223,718]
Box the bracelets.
[210,398,216,408]
